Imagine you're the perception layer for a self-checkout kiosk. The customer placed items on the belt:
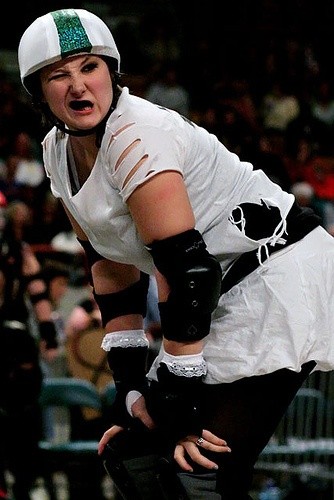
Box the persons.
[19,9,333,499]
[0,0,334,499]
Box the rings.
[196,437,203,445]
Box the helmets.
[17,9,120,97]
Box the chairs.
[38,370,334,500]
